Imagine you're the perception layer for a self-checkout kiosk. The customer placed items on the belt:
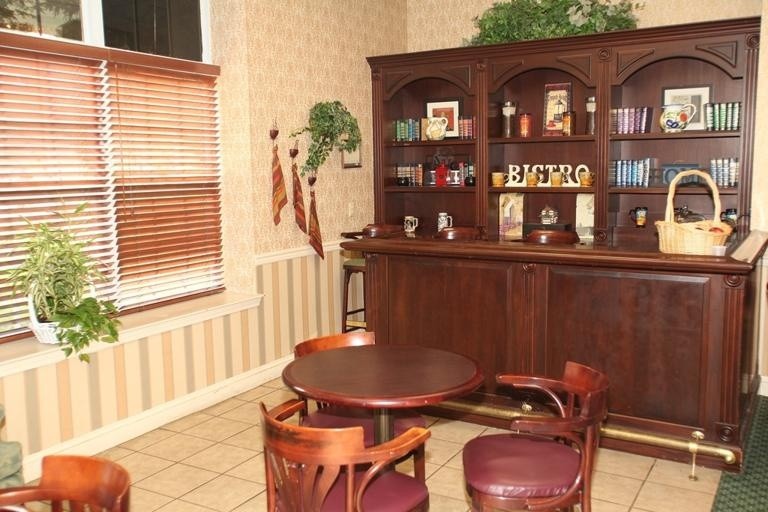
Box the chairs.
[257,395,433,512]
[339,231,366,333]
[1,452,132,512]
[293,331,426,449]
[459,359,614,511]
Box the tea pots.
[424,116,449,140]
[658,102,698,134]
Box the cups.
[720,208,738,233]
[436,212,454,235]
[577,171,597,188]
[628,205,648,230]
[403,215,419,236]
[490,172,509,188]
[549,171,569,187]
[524,171,544,186]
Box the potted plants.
[6,192,124,364]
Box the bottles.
[585,97,597,135]
[501,101,516,139]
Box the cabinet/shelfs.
[541,81,573,137]
[364,46,480,230]
[603,15,762,227]
[481,33,603,231]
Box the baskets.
[654,170,734,256]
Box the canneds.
[562,111,576,136]
[519,113,532,137]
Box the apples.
[709,227,723,234]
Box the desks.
[363,228,767,476]
[281,341,486,476]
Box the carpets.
[709,394,768,511]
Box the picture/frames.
[660,83,714,134]
[423,95,465,140]
